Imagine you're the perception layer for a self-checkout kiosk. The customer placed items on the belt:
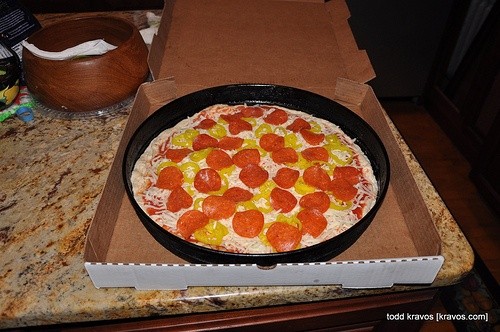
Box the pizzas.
[130,103,379,257]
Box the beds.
[0,1,474,332]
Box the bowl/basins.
[23,14,152,112]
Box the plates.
[28,93,133,120]
[123,84,390,267]
